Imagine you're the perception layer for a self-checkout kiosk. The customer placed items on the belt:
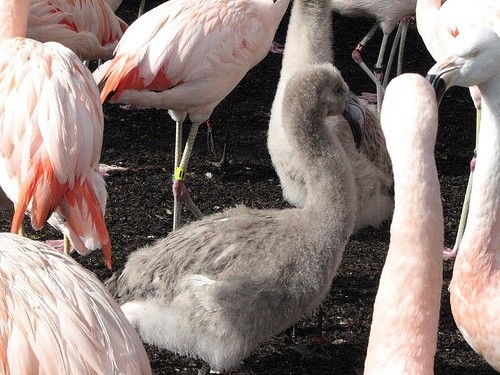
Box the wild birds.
[0,0,500,375]
